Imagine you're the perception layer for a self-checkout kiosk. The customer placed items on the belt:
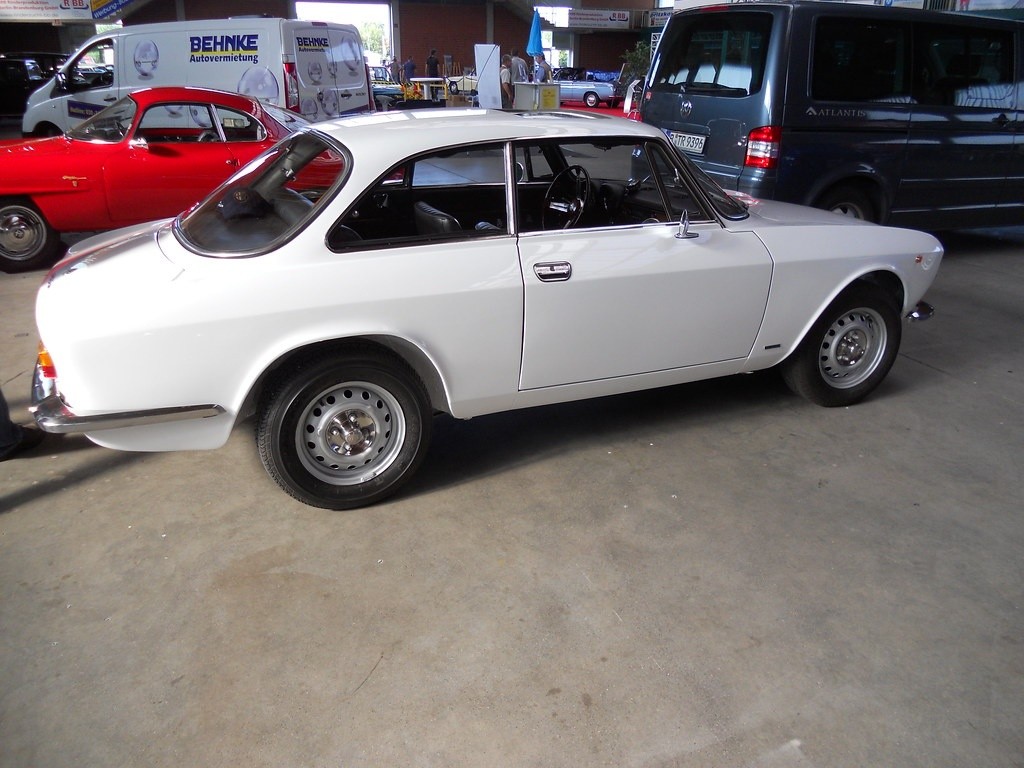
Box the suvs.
[0,53,114,121]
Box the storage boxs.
[445,95,471,108]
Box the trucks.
[20,14,377,124]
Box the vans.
[629,1,1024,226]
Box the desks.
[410,78,445,100]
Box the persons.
[390,59,402,80]
[535,53,551,82]
[425,50,440,102]
[402,56,415,82]
[511,49,529,86]
[500,54,512,104]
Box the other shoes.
[432,99,435,103]
[433,93,438,100]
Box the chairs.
[587,75,594,82]
[414,200,461,235]
[476,222,500,230]
[940,54,1008,107]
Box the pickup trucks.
[548,67,625,108]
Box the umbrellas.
[526,10,543,100]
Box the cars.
[0,87,406,273]
[445,66,478,96]
[368,65,405,107]
[29,105,941,510]
